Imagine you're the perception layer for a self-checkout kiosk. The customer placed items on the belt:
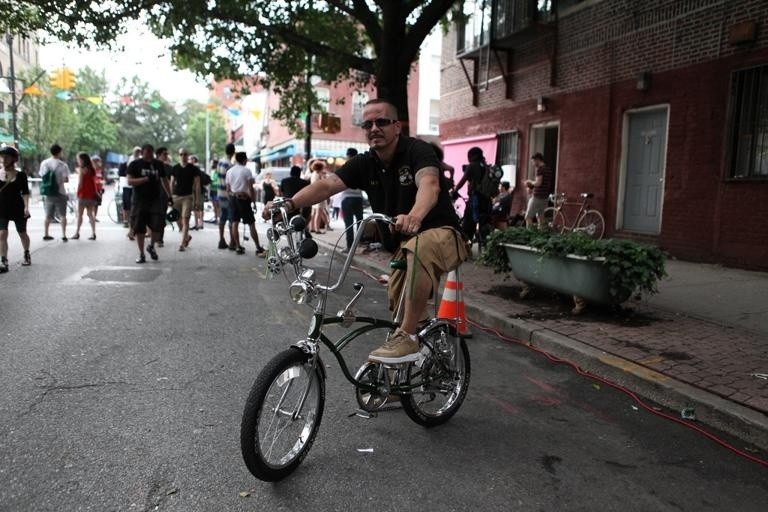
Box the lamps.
[537,96,548,112]
[636,72,650,90]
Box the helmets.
[0,146,18,161]
[166,206,179,221]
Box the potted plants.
[477,224,669,314]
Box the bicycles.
[108,185,125,224]
[53,188,78,225]
[239,194,476,482]
[537,189,606,242]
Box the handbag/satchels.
[95,191,101,205]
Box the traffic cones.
[434,258,476,344]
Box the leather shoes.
[43,233,96,241]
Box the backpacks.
[470,163,502,198]
[39,159,63,197]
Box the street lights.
[5,11,25,172]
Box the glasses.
[360,117,397,129]
[179,153,186,156]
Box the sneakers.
[309,225,334,234]
[0,256,8,272]
[135,255,145,262]
[362,394,401,408]
[341,248,356,253]
[146,244,157,259]
[368,327,419,364]
[21,250,30,266]
[367,245,376,251]
[128,219,264,254]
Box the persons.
[440,147,512,254]
[262,158,342,235]
[38,145,69,241]
[523,153,552,229]
[118,145,206,263]
[1,148,31,273]
[72,153,103,240]
[340,148,365,253]
[261,99,468,366]
[211,144,266,253]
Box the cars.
[105,167,119,184]
